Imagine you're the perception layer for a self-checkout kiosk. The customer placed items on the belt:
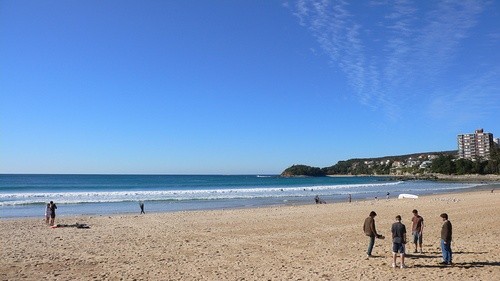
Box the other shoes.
[419,250,422,254]
[414,249,417,253]
[367,253,371,257]
[438,262,452,265]
[378,234,382,238]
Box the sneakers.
[392,263,396,268]
[401,265,408,268]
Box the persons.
[391,215,407,268]
[438,213,452,265]
[411,209,424,254]
[362,211,385,257]
[45,201,57,226]
[139,201,145,214]
